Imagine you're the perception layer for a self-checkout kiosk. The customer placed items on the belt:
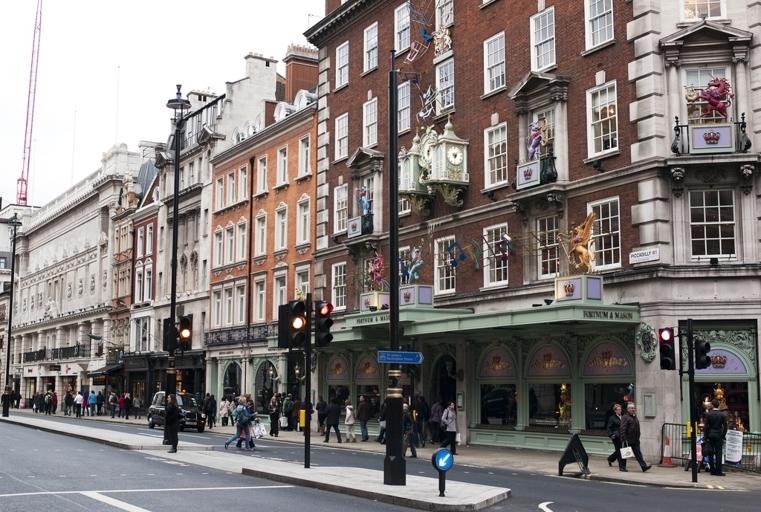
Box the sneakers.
[429,439,435,444]
[421,440,425,448]
[225,440,228,450]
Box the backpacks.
[46,394,52,405]
[111,393,118,404]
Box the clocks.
[447,146,464,166]
[419,125,438,196]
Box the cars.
[482,386,512,418]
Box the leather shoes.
[642,465,651,472]
[619,467,628,472]
[608,458,612,467]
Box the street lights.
[2,211,22,417]
[163,82,191,453]
[382,47,421,486]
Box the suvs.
[147,390,206,434]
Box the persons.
[702,398,728,477]
[162,393,180,453]
[402,394,458,457]
[620,401,652,472]
[606,403,628,472]
[195,390,386,451]
[1,388,141,419]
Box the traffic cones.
[659,437,677,467]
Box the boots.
[345,437,356,443]
[319,424,326,436]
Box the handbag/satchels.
[619,439,636,460]
[237,412,250,427]
[440,420,447,432]
[279,416,288,428]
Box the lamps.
[86,333,118,348]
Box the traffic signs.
[377,351,425,365]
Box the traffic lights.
[659,326,676,371]
[314,299,335,349]
[179,314,194,351]
[695,337,712,370]
[288,298,309,350]
